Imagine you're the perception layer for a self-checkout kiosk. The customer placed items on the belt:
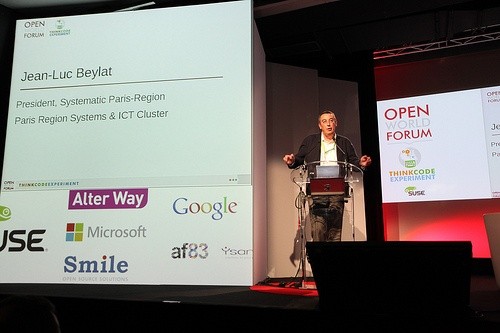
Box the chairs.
[305,240,470,333]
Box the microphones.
[332,137,347,155]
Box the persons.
[282,110,373,241]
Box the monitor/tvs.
[302,238,474,309]
